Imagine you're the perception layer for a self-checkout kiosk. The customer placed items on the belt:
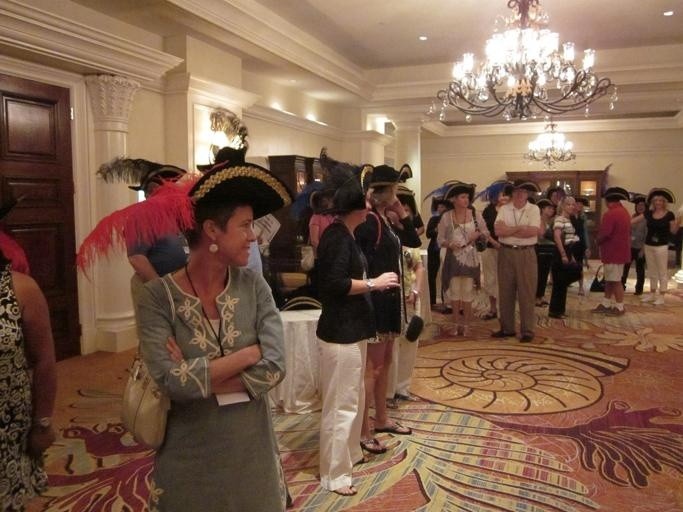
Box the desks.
[270,305,325,419]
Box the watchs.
[32,416,54,428]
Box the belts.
[497,240,535,250]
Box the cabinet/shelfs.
[504,165,608,260]
[268,152,322,272]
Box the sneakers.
[653,297,665,305]
[640,296,657,303]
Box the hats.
[196,146,248,172]
[600,187,676,206]
[128,162,188,192]
[430,178,590,213]
[309,163,416,216]
[188,160,295,221]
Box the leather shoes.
[491,330,516,338]
[519,334,535,343]
[591,304,612,313]
[463,324,473,338]
[448,322,459,337]
[605,307,626,317]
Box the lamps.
[428,0,616,131]
[522,120,575,169]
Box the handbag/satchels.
[590,264,607,293]
[121,348,173,451]
[405,314,424,343]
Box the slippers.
[333,392,417,496]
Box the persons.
[1,247,59,511]
[135,159,293,512]
[128,166,188,332]
